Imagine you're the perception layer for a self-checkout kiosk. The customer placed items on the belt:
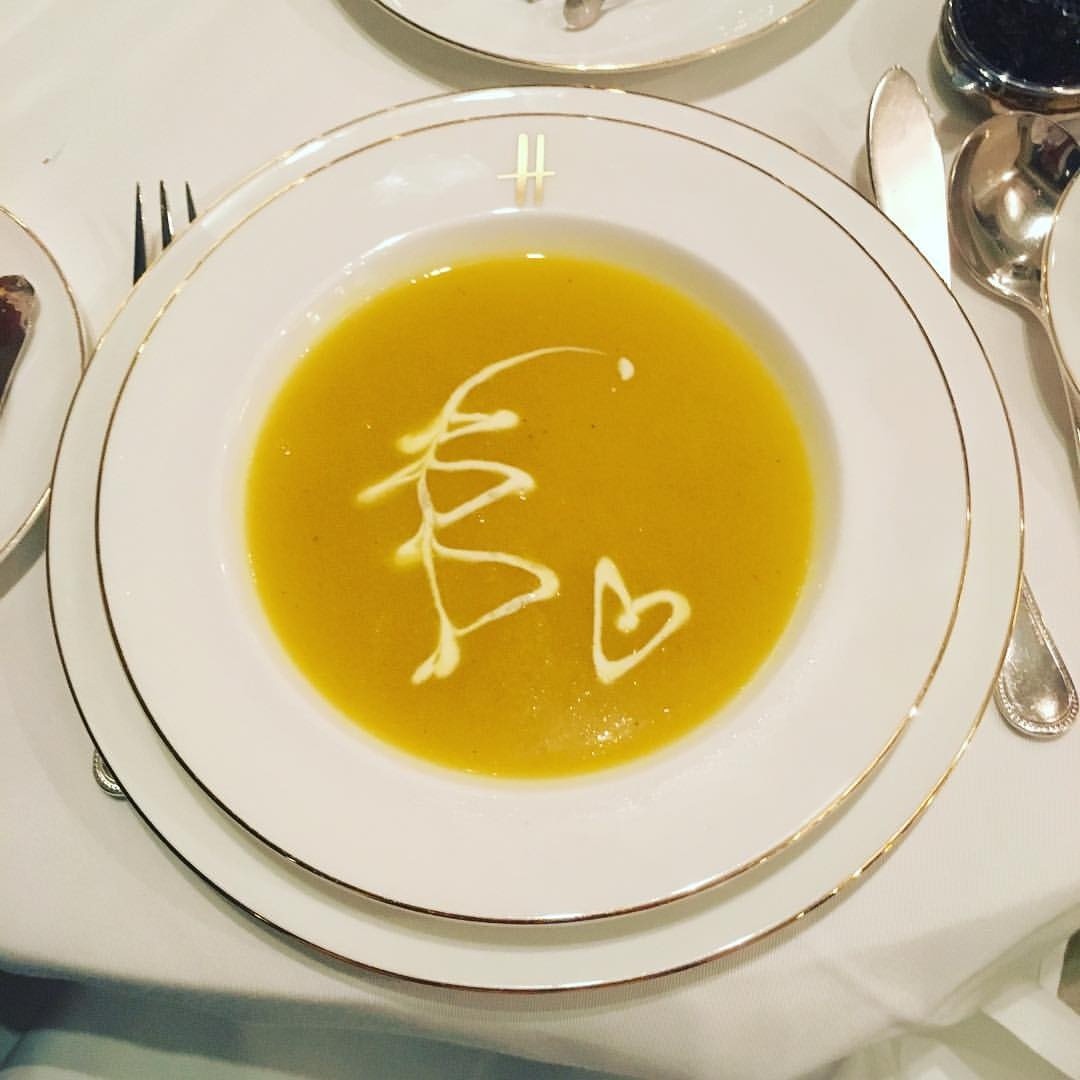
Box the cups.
[937,0,1079,122]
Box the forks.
[92,169,196,799]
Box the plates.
[371,1,818,79]
[1044,163,1080,392]
[3,204,87,569]
[42,77,1023,994]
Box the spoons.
[859,54,1080,733]
